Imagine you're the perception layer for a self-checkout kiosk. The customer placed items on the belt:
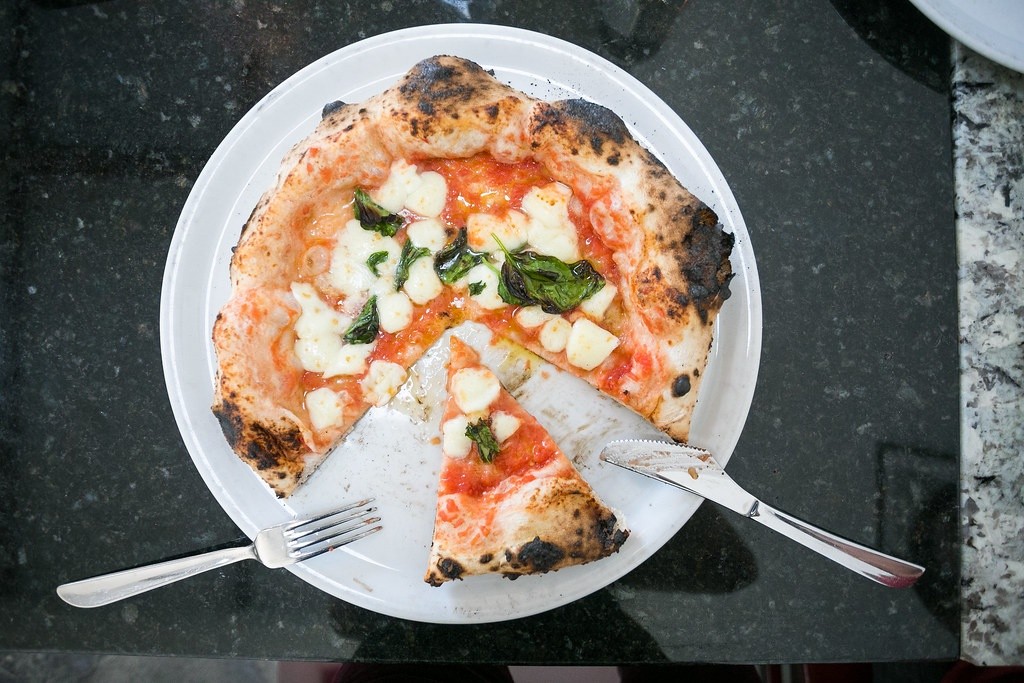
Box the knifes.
[597,438,927,597]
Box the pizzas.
[210,54,736,587]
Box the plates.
[160,20,761,625]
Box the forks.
[57,499,386,609]
